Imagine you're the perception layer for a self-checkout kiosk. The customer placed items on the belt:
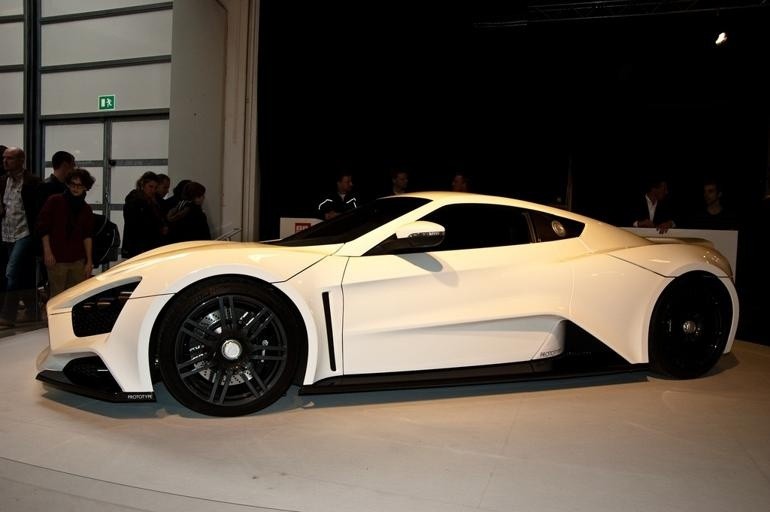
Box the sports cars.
[35,188,744,418]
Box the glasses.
[69,182,85,188]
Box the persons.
[657,181,737,229]
[392,171,409,192]
[622,177,671,229]
[0,145,96,325]
[316,173,359,220]
[449,173,470,192]
[122,171,210,259]
